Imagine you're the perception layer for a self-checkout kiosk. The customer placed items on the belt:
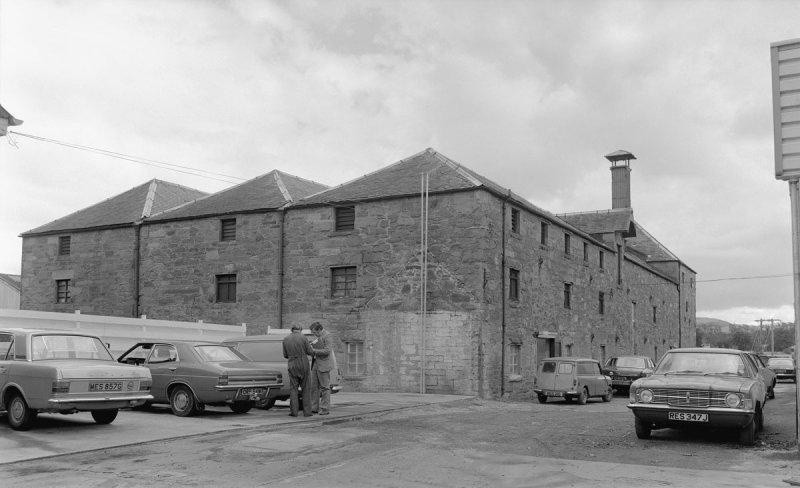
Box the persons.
[307,321,333,416]
[282,323,315,417]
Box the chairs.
[158,348,170,358]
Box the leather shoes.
[304,410,329,416]
[289,413,295,416]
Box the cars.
[0,327,154,432]
[626,347,777,445]
[116,340,280,416]
[766,357,795,381]
[602,354,656,396]
[534,356,612,405]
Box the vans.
[221,335,339,407]
